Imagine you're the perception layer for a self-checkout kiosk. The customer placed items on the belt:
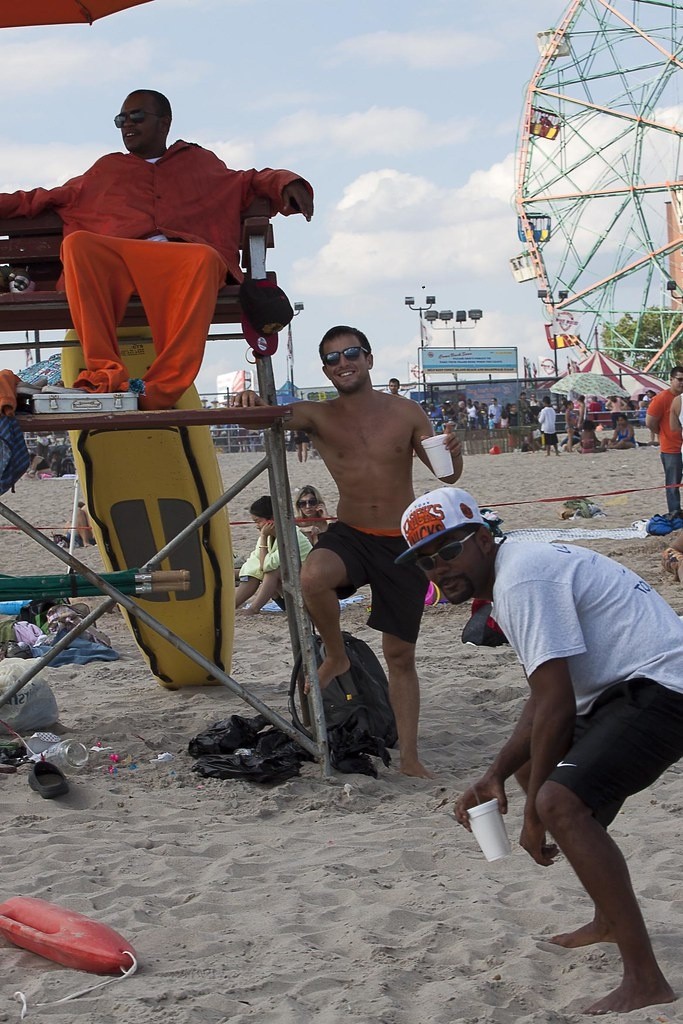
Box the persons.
[216,424,263,453]
[294,432,309,463]
[646,367,683,510]
[0,89,315,404]
[420,392,541,453]
[389,378,405,399]
[64,502,94,548]
[538,390,660,456]
[234,484,334,615]
[28,431,66,477]
[229,325,463,780]
[394,487,683,1016]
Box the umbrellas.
[0,0,155,28]
[549,372,630,398]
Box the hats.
[250,496,274,519]
[239,280,295,358]
[395,488,484,565]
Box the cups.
[421,433,455,480]
[39,738,89,775]
[466,796,512,864]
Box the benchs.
[0,165,272,327]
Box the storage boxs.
[14,391,140,414]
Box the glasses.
[324,345,369,366]
[416,525,481,571]
[672,376,683,382]
[114,111,165,128]
[298,499,318,509]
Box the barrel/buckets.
[489,445,500,454]
[425,580,441,607]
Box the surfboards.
[61,325,236,694]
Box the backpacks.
[290,634,399,748]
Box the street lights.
[286,301,304,396]
[404,295,436,405]
[425,309,482,401]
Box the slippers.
[28,761,67,798]
